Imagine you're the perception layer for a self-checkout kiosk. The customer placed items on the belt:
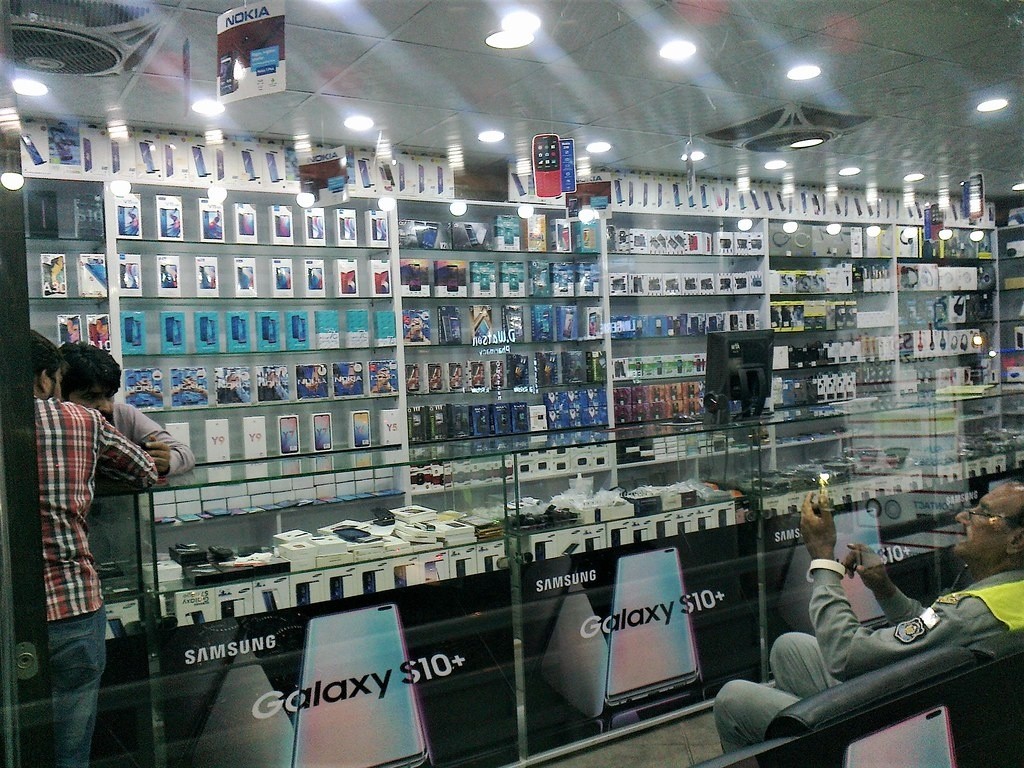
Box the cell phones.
[22,125,764,462]
[109,506,740,768]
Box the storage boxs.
[28,189,1024,639]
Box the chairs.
[763,627,1024,768]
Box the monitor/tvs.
[703,329,775,420]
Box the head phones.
[951,336,958,349]
[960,334,968,351]
[954,296,965,316]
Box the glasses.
[961,502,1013,521]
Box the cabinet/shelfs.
[19,174,1024,561]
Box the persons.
[713,481,1024,768]
[29,326,159,768]
[53,342,196,565]
